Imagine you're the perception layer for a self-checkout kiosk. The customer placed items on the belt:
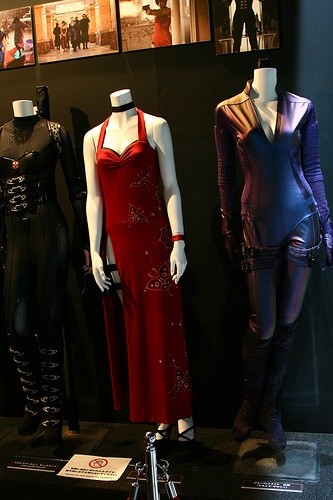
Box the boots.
[259,317,302,451]
[25,343,64,447]
[9,346,42,436]
[232,320,279,443]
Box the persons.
[145,0,172,48]
[9,16,29,56]
[214,69,333,451]
[83,89,194,444]
[228,0,260,53]
[53,14,91,52]
[0,100,92,439]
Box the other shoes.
[174,424,196,457]
[153,424,172,446]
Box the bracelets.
[172,233,185,242]
